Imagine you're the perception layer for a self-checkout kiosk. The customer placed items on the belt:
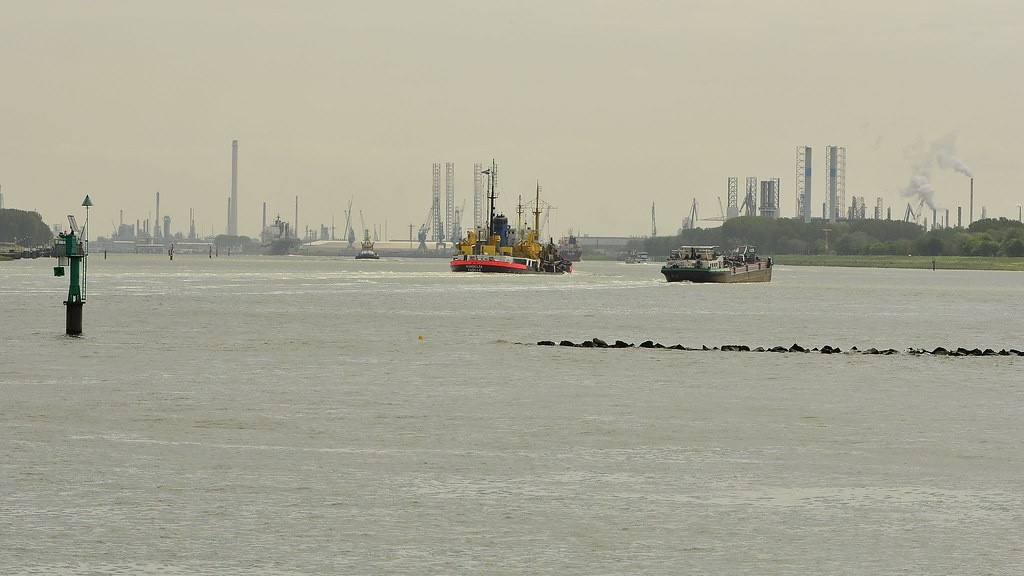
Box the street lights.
[822,229,832,255]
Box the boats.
[625,250,650,264]
[354,229,380,259]
[660,245,773,283]
[449,159,572,275]
